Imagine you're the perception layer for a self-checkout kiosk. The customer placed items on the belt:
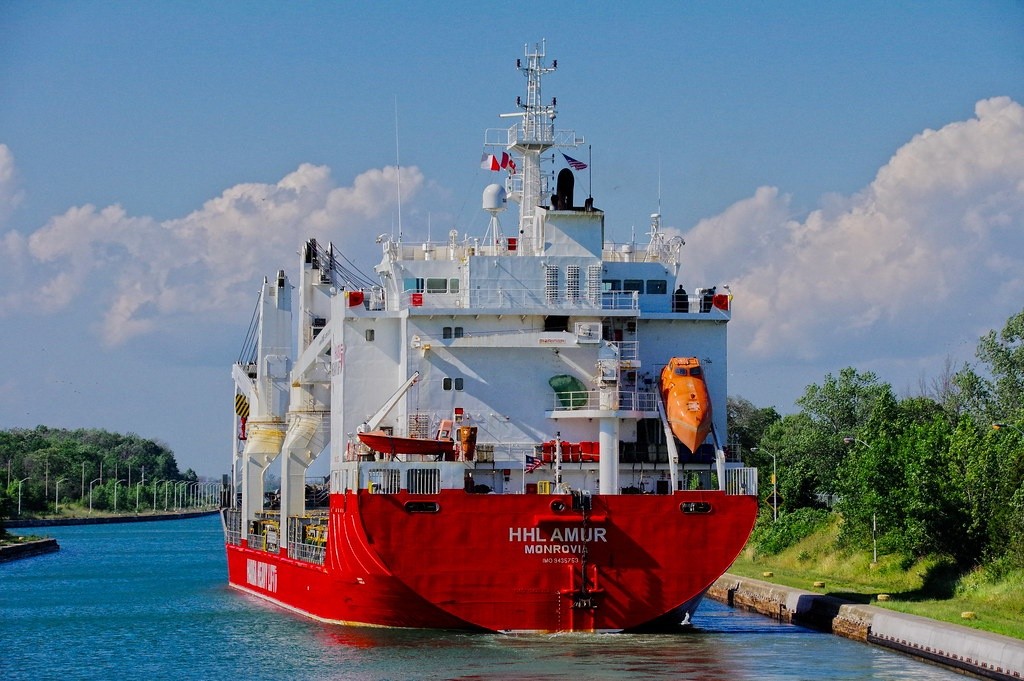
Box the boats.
[357,430,453,455]
[217,36,762,636]
[658,356,712,454]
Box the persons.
[675,285,686,294]
[696,482,705,490]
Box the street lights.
[166,479,175,510]
[154,479,165,509]
[90,478,102,512]
[750,447,777,522]
[56,478,68,513]
[137,479,147,508]
[114,479,126,509]
[18,477,33,514]
[174,480,223,510]
[843,437,877,562]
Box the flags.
[525,456,546,474]
[499,152,517,175]
[481,153,499,171]
[562,153,587,171]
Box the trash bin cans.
[542,440,599,463]
